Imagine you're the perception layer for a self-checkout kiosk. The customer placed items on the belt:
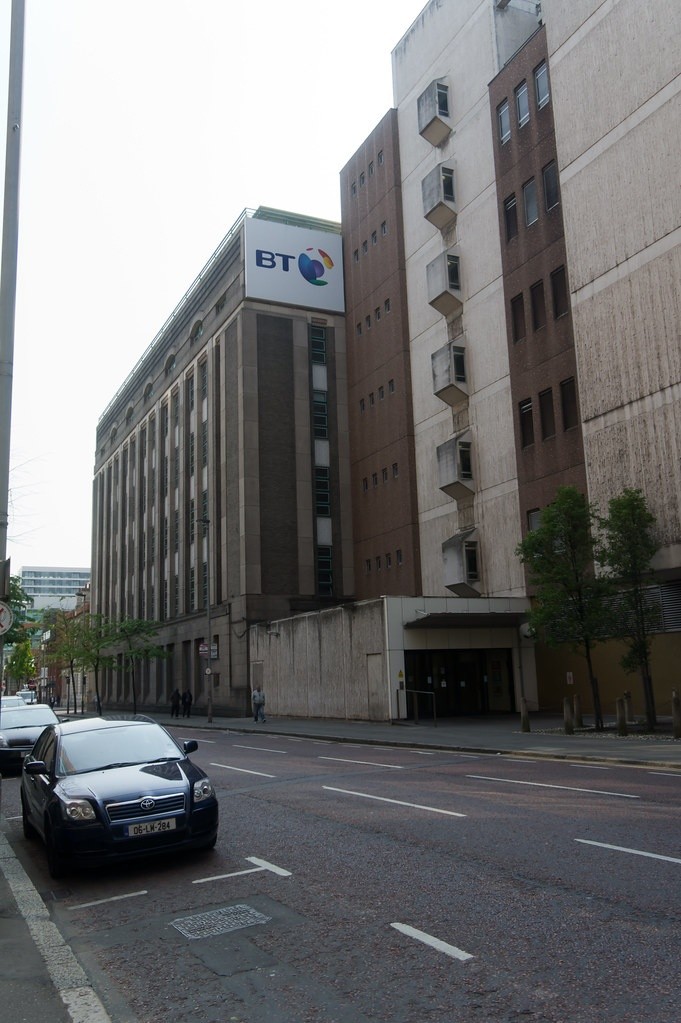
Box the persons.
[251,684,266,724]
[92,695,102,714]
[50,695,55,709]
[171,688,181,719]
[182,687,193,718]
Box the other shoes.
[262,719,266,723]
[254,721,257,724]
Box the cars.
[0,695,26,706]
[0,703,70,770]
[20,713,219,880]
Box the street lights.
[195,518,212,723]
[75,592,86,715]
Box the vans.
[16,690,38,705]
